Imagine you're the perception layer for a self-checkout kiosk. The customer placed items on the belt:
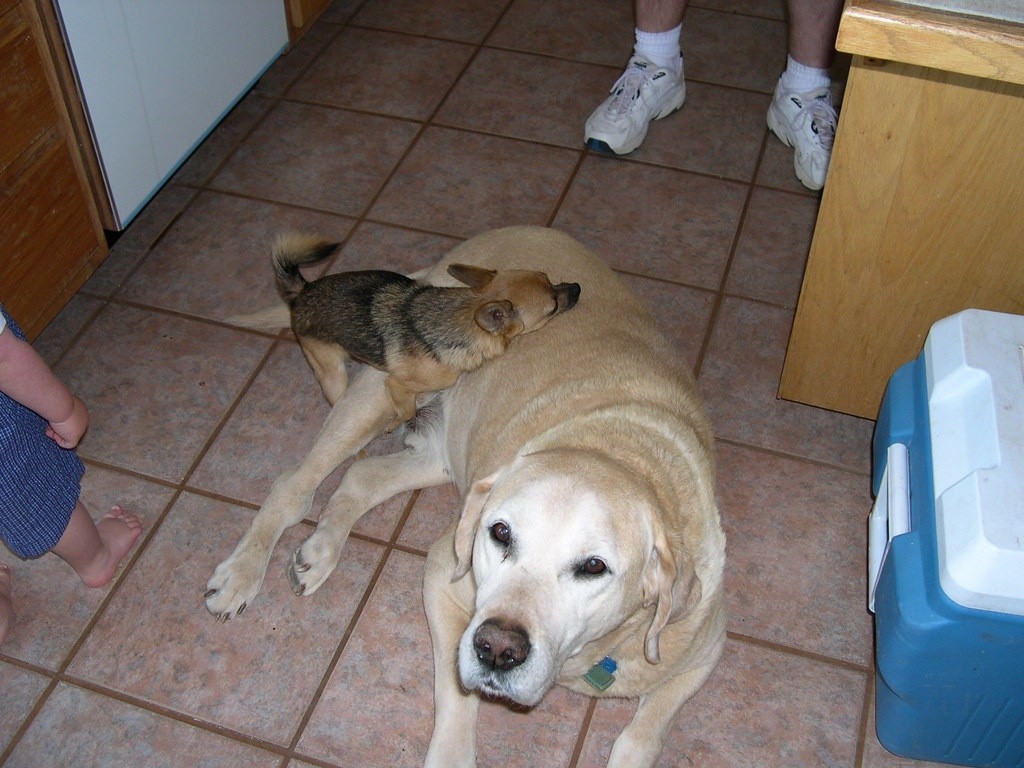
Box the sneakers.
[766,76,843,190]
[583,52,686,156]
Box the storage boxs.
[869,310,1024,768]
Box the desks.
[776,0,1024,421]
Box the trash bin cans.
[855,308,1024,768]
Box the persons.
[583,0,847,193]
[0,313,144,644]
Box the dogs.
[270,230,582,461]
[204,225,729,768]
